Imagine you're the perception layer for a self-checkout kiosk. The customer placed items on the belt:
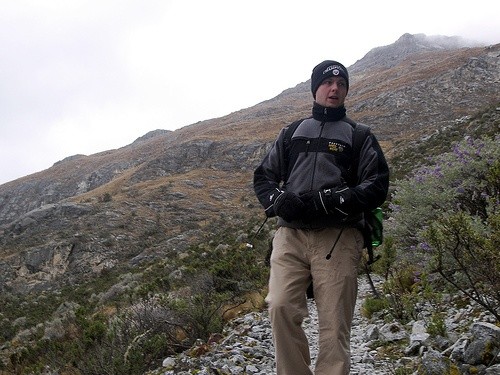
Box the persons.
[253,60,390,375]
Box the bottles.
[372,207,383,246]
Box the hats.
[311,60,349,100]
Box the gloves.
[270,187,304,223]
[298,185,362,229]
